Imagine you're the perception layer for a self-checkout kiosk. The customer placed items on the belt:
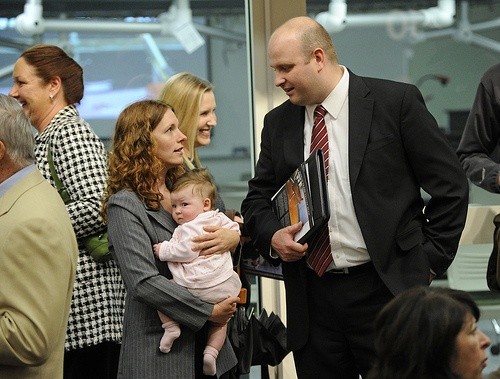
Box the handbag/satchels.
[83,229,112,263]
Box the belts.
[329,263,372,274]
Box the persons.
[9,44,127,379]
[0,93,79,379]
[240,16,471,379]
[456,63,500,294]
[160,73,245,225]
[369,286,491,379]
[103,98,241,379]
[153,168,242,375]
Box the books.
[271,149,330,245]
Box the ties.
[309,106,332,277]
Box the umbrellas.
[226,288,292,376]
